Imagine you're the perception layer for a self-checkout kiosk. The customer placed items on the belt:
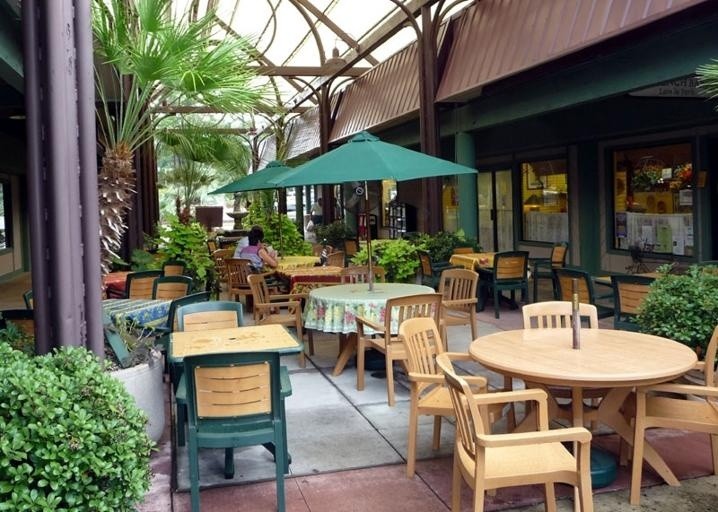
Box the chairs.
[0,290,36,353]
[204,239,279,313]
[247,275,315,357]
[615,317,717,505]
[448,241,570,319]
[354,291,444,408]
[105,260,193,299]
[417,249,465,284]
[312,236,360,267]
[521,298,602,437]
[432,268,480,351]
[433,348,596,511]
[339,267,391,357]
[398,312,518,482]
[173,298,245,446]
[551,267,662,320]
[179,347,291,511]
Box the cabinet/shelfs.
[386,198,415,240]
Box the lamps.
[523,191,542,209]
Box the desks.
[302,283,442,378]
[101,299,171,334]
[271,252,344,288]
[593,265,706,308]
[464,326,698,491]
[165,320,304,478]
[447,250,504,311]
[99,270,132,300]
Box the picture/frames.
[526,162,548,190]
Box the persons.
[241,229,279,313]
[332,197,341,221]
[232,226,265,257]
[309,197,323,240]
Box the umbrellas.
[208,159,296,260]
[267,130,480,370]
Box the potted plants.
[99,311,166,448]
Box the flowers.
[631,159,695,192]
